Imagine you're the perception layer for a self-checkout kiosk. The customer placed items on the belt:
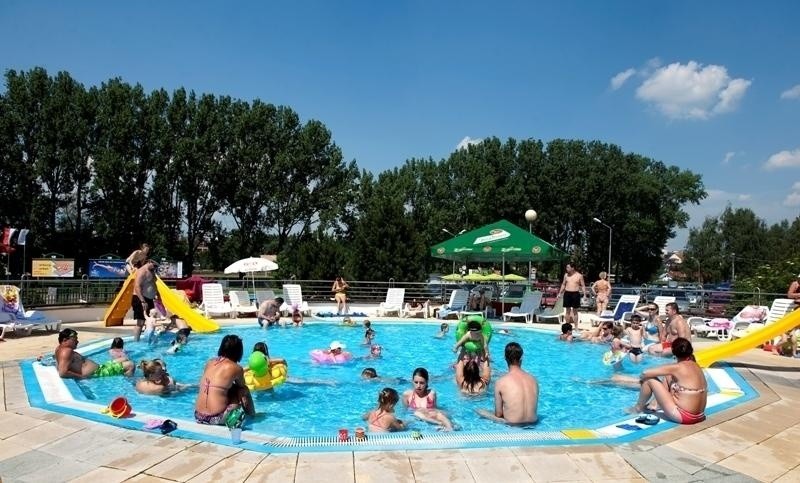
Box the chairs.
[379,287,405,318]
[459,290,492,320]
[229,290,258,320]
[502,290,544,324]
[282,284,312,317]
[731,297,795,351]
[535,293,566,324]
[436,290,470,320]
[621,294,676,329]
[589,294,640,327]
[687,303,770,342]
[0,284,62,341]
[254,289,288,318]
[199,282,234,320]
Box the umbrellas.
[430,219,571,321]
[224,257,279,304]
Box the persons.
[437,323,449,338]
[360,369,414,389]
[624,316,648,362]
[143,309,168,343]
[362,389,409,433]
[361,319,371,332]
[453,322,490,360]
[788,275,800,359]
[136,360,201,398]
[290,304,304,327]
[331,278,350,315]
[124,244,149,275]
[165,334,188,355]
[592,272,611,316]
[361,330,374,344]
[55,329,136,379]
[467,290,490,311]
[356,344,383,361]
[634,304,663,352]
[647,303,692,358]
[242,344,338,387]
[555,263,587,332]
[258,298,284,330]
[163,316,190,342]
[473,343,539,427]
[599,322,615,343]
[131,255,161,343]
[608,325,633,370]
[456,346,491,396]
[195,334,255,426]
[308,342,346,367]
[624,338,707,425]
[402,368,454,432]
[558,324,597,343]
[109,337,133,359]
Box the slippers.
[644,415,660,425]
[148,418,163,429]
[636,414,647,423]
[159,419,171,427]
[162,422,177,434]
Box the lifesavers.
[456,314,492,352]
[310,348,350,364]
[242,351,287,391]
[603,334,632,366]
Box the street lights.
[442,228,467,274]
[593,217,612,283]
[690,256,701,290]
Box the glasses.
[68,331,78,338]
[647,309,655,311]
[151,262,158,268]
[603,328,607,330]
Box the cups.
[231,428,242,444]
[338,429,348,443]
[354,427,365,443]
[412,424,421,439]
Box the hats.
[330,341,347,350]
